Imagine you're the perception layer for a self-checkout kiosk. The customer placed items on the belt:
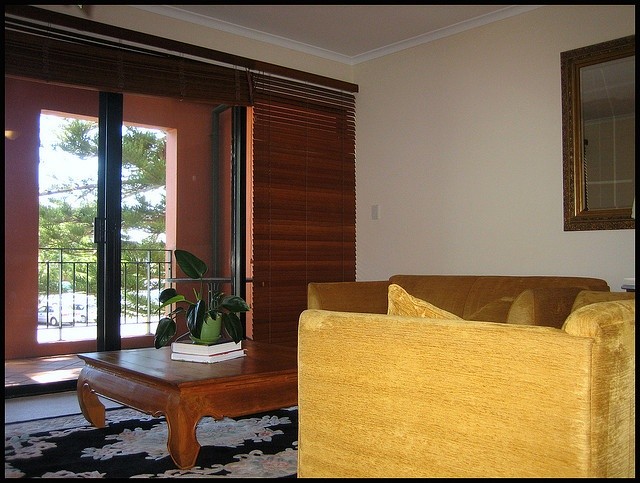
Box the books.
[170,348,247,364]
[171,340,241,356]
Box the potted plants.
[152,249,250,349]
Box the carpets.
[4,404,299,477]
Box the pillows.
[387,283,463,319]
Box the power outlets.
[371,205,379,220]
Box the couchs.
[307,276,609,330]
[297,291,636,476]
